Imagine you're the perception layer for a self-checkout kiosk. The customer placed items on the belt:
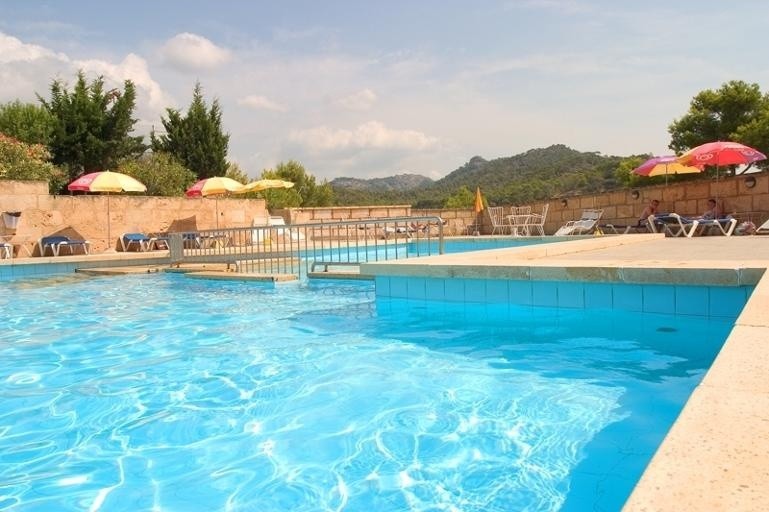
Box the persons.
[701,200,719,219]
[638,200,662,233]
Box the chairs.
[553,208,605,236]
[646,213,738,238]
[120,232,202,251]
[487,203,549,237]
[39,235,87,257]
[0,243,13,259]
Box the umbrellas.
[68,169,147,248]
[185,175,245,246]
[243,178,295,245]
[677,140,768,228]
[473,185,484,231]
[629,157,701,211]
